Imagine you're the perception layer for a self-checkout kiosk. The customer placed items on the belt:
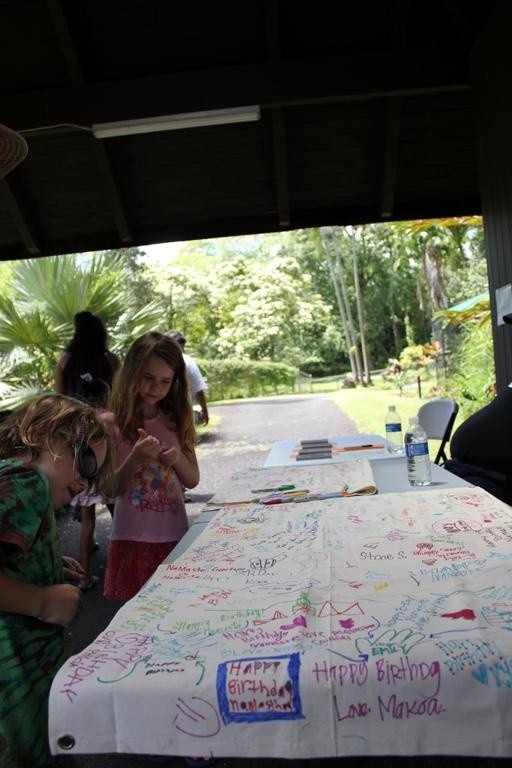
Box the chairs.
[407,399,463,469]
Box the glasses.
[59,431,98,487]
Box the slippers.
[76,573,99,590]
[90,541,101,553]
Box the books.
[297,439,332,461]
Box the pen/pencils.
[251,484,348,504]
[335,444,385,451]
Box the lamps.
[90,105,263,140]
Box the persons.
[88,330,200,610]
[162,330,208,503]
[55,314,122,552]
[1,392,110,767]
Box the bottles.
[384,405,405,455]
[403,415,432,487]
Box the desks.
[50,456,511,766]
[264,434,406,466]
